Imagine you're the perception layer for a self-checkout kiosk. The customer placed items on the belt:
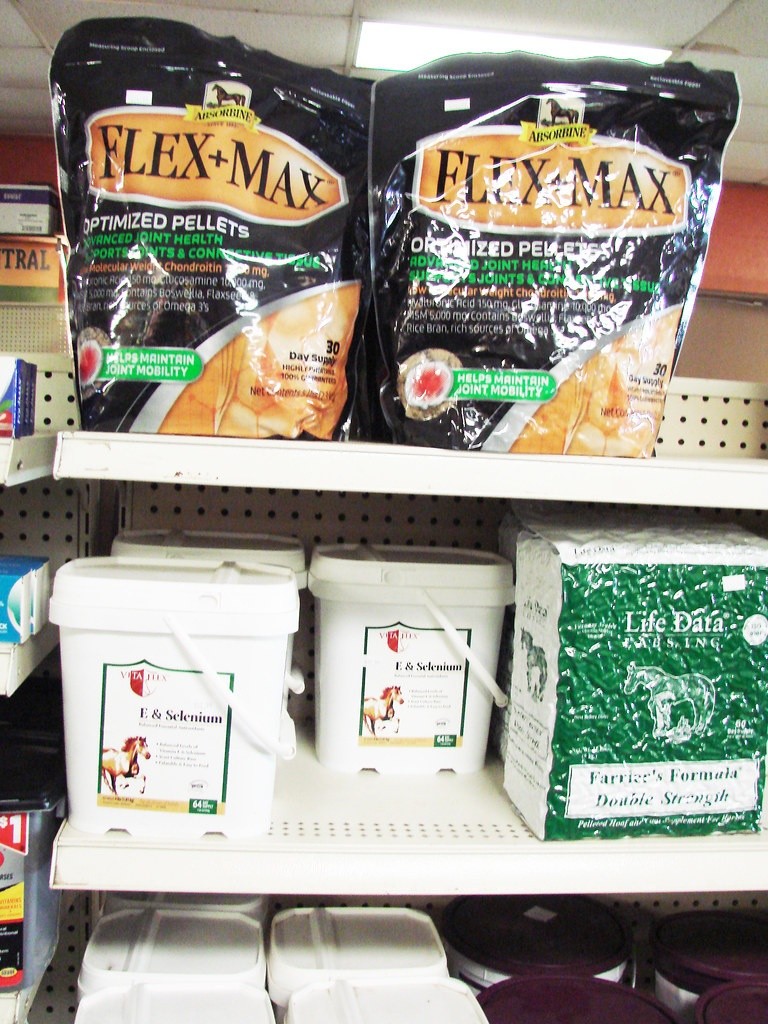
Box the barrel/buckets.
[47,527,515,842]
[74,892,768,1024]
[0,746,64,993]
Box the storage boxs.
[0,357,38,440]
[0,555,51,645]
[0,183,62,236]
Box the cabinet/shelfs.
[50,426,768,1024]
[0,177,105,1024]
